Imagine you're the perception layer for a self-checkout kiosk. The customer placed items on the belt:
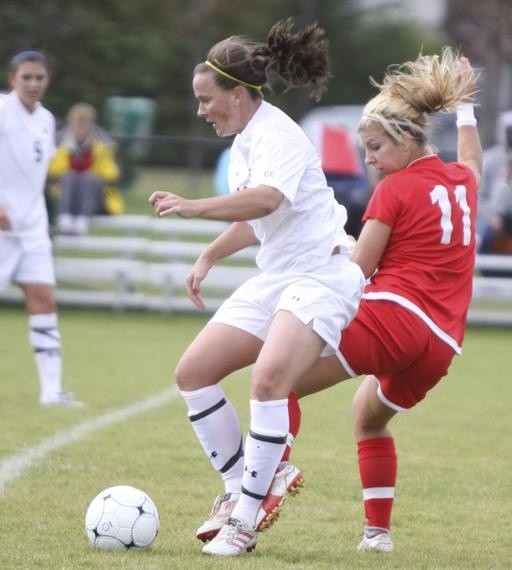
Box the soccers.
[86,485,159,551]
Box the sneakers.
[356,533,395,554]
[53,215,87,237]
[196,463,305,557]
[38,386,83,408]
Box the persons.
[256,43,483,554]
[148,17,366,557]
[44,101,123,235]
[477,112,512,254]
[0,49,85,408]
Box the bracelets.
[455,103,476,129]
